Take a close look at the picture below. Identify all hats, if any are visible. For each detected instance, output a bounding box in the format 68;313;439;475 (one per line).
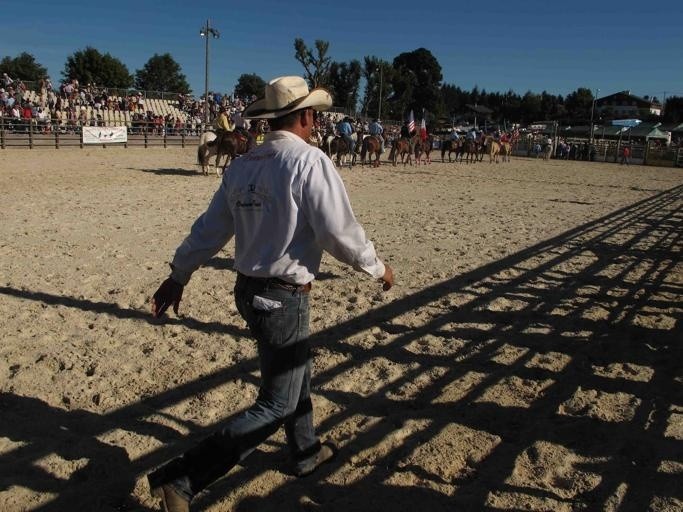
241;75;333;120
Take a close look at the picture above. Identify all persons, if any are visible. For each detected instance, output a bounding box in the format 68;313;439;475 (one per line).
150;75;396;509
1;74;270;153
305;110;682;166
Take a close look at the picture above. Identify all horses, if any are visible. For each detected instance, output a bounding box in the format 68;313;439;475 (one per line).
308;125;324;147
197;119;258;179
440;137;465;163
359;132;389;169
466;133;487;165
387;134;413;168
489;133;512;165
542;138;553;161
414;135;434;165
323;130;361;170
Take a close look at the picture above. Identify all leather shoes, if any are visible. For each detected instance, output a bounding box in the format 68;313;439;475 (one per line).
146;471;189;512
294;439;338;476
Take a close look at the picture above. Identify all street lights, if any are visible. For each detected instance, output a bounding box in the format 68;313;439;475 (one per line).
200;18;219;131
372;59;388;119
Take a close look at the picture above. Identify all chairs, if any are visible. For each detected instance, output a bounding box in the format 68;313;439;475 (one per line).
21;90;186;128
